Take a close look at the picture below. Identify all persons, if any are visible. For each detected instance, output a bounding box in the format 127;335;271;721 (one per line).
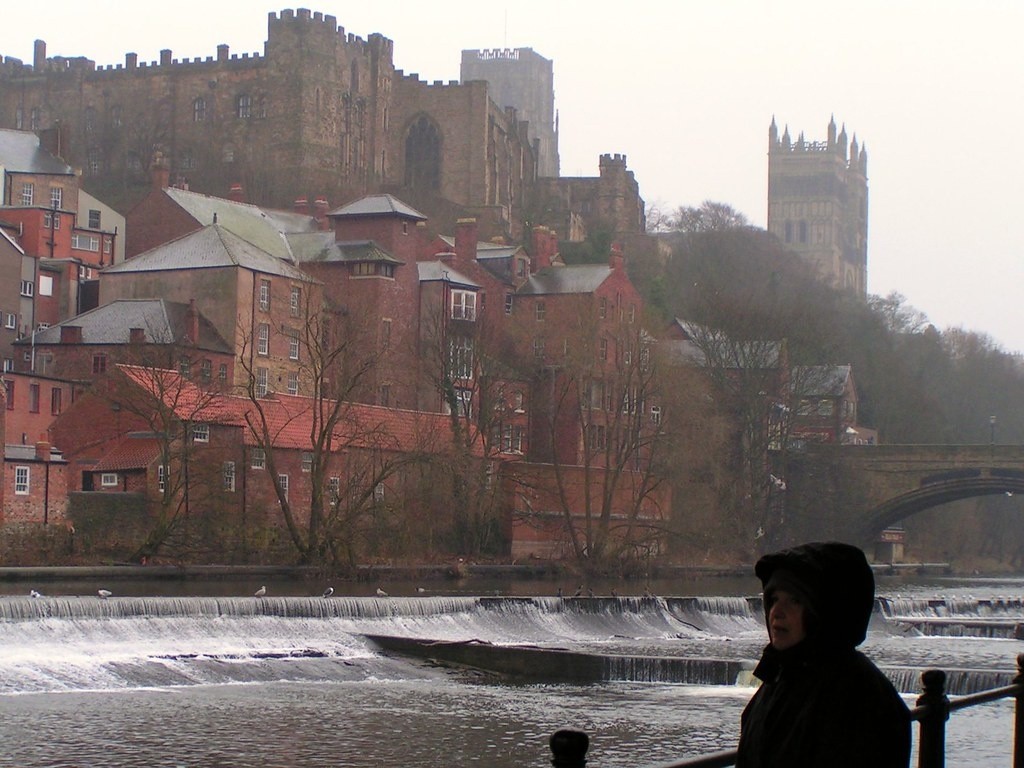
735;541;912;768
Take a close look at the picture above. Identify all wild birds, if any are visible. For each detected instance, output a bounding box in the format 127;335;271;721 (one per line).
969;596;973;600
557;589;564;597
415;588;425;592
323;586;334;598
255;586;266;598
30;589;41;599
897;594;900;599
612;589;620;597
588;588;594;596
910;596;914;599
990;595;1021;600
941;596;945;599
952;595;956;600
377;587;390;598
962;595;966;598
574;584;583;596
98;590;111;599
